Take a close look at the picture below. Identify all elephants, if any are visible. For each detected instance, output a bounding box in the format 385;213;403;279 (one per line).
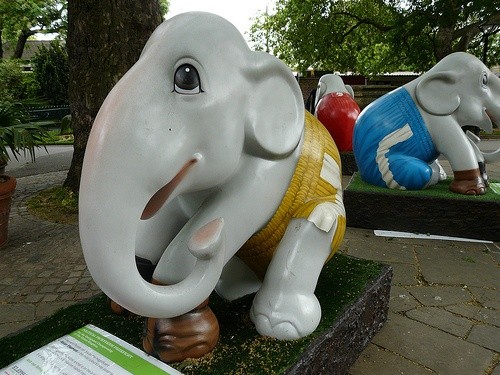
79;11;346;363
353;52;500;195
314;74;360;152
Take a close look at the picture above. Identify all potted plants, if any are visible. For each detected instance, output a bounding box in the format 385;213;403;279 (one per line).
1;93;65;250
